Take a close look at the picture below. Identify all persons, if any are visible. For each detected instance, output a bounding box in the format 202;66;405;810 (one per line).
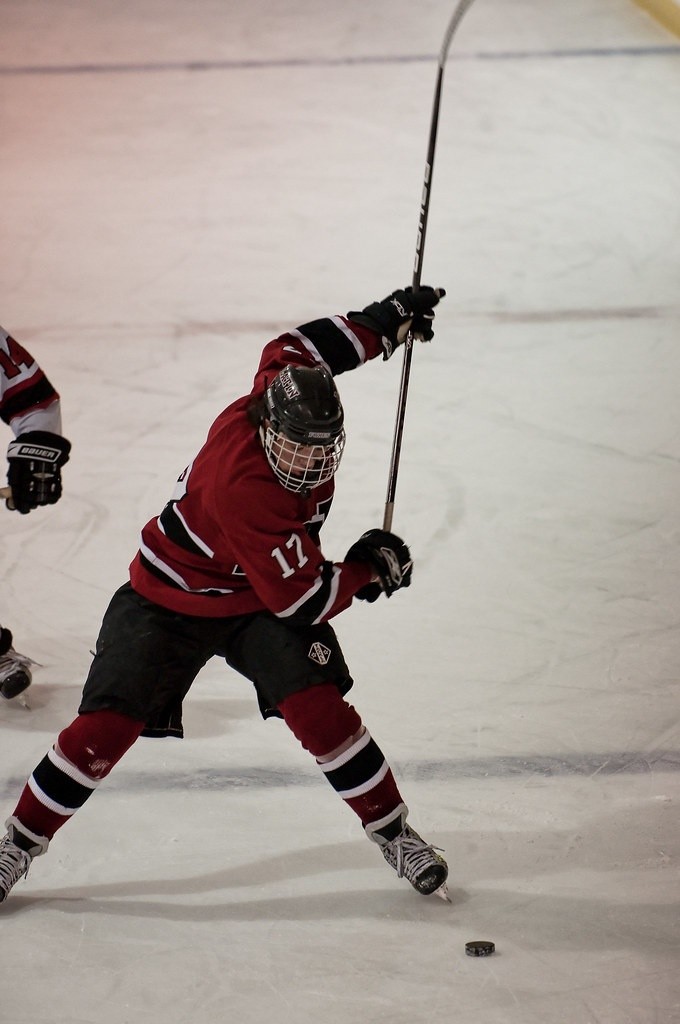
1;284;450;903
0;328;73;710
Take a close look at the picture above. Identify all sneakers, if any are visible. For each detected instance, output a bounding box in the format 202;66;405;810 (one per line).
361;802;452;903
0;817;49;905
0;626;46;713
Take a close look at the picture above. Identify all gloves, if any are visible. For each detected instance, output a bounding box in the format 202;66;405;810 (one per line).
6;430;73;515
344;528;413;603
347;281;446;361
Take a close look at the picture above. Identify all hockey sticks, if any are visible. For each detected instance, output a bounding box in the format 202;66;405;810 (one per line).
383;1;474;532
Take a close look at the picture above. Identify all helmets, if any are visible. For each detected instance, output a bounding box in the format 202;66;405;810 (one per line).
263;364;344;494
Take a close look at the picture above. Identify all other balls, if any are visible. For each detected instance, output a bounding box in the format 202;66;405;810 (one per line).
464;940;496;958
